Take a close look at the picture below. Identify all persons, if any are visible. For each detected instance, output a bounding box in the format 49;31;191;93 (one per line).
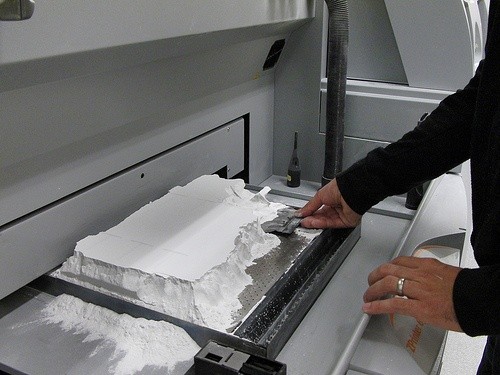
295;0;500;375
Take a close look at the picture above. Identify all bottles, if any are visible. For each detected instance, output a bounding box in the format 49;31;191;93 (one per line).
287;131;301;188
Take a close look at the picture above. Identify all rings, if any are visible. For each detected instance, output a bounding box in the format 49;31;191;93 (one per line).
398;276;406;295
